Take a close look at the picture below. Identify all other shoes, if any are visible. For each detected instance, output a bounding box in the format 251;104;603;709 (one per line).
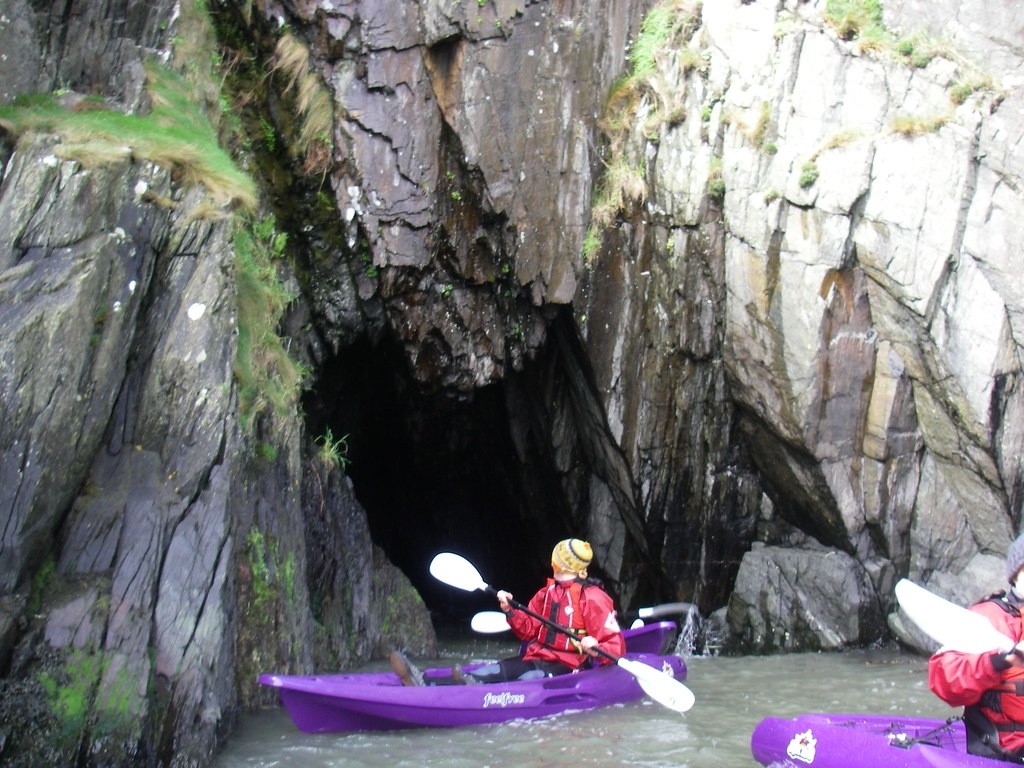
391;651;431;686
453;663;483;686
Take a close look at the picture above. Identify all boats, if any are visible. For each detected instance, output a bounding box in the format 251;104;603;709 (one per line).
257;601;700;731
750;711;1024;768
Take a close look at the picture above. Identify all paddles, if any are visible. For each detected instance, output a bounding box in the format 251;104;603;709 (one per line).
470;600;699;634
894;576;1018;656
429;553;697;714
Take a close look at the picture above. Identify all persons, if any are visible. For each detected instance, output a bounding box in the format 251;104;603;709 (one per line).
927;532;1024;763
389;539;625;685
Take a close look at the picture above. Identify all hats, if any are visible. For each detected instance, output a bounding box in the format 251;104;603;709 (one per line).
1006;534;1024;586
552;539;593;579
